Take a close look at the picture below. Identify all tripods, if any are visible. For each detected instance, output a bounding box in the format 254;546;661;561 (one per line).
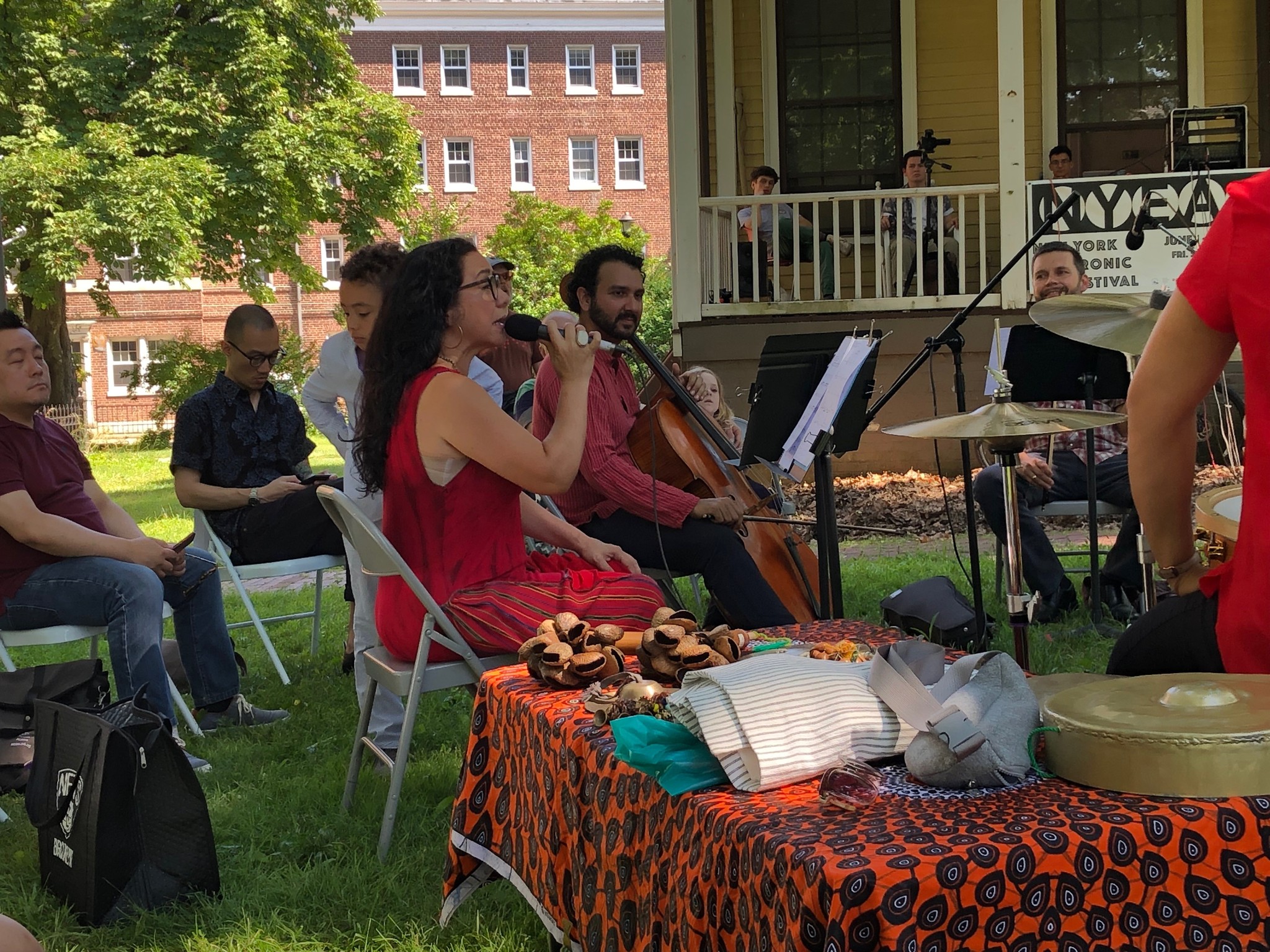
903;150;961;296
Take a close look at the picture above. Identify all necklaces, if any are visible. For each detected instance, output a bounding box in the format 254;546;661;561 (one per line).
439;354;459;370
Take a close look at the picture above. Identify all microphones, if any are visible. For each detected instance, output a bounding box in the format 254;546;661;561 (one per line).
505;314;626;358
1125;190;1152;250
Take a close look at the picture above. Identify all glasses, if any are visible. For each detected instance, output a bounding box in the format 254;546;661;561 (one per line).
227;340;286;368
495;272;514;281
457;274;501;300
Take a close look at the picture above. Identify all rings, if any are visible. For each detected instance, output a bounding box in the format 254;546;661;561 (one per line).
1032;475;1037;479
576;331;589;346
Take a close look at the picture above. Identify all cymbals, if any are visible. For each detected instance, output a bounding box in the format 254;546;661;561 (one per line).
880;392;1128;440
1029;288;1244;362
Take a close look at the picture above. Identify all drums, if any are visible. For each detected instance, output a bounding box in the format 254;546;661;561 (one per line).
1195;480;1244;569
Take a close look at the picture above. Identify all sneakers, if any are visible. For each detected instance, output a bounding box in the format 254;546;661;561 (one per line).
174;735;212;773
199;694;289;731
826;233;852;257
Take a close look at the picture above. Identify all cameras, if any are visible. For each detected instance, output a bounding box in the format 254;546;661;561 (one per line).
916;129;952;151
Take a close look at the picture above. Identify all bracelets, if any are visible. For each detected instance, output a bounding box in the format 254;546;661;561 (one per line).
1157;546;1204;579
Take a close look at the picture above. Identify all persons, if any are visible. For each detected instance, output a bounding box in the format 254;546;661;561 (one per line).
168;304;355;675
301;237;794;759
1049;145;1076;179
880;150;960;298
971;241;1153;625
0;308;291;773
1105;170;1270;678
737;165;853;300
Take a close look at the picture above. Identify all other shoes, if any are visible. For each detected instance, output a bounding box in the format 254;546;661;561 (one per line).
1030;581;1079;626
342;641;355;675
1099;576;1143;622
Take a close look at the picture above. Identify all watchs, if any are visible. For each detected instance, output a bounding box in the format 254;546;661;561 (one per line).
248;487;261;509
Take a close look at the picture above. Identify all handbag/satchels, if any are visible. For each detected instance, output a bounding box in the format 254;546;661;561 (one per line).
876;574;997;651
24;680;226;929
864;631;1039;787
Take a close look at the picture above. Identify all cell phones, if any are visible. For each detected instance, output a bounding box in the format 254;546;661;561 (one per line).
299;475;330;485
166;532;196;560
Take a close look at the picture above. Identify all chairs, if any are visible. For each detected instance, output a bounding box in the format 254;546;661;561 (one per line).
191;508;345;685
744;227;794;301
996;499;1132;606
0;600;205;745
316;486;523;862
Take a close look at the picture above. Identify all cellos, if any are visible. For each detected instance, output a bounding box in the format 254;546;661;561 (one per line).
625;329;833;630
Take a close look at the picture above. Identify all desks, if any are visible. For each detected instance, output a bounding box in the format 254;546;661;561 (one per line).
440;621;1270;952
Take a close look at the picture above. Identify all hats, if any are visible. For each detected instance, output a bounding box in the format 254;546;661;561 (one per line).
486;257;514;271
